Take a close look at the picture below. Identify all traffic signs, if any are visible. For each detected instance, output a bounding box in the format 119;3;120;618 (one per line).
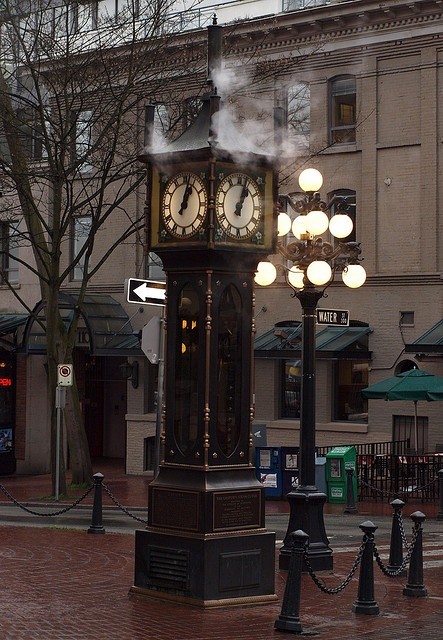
315;308;350;327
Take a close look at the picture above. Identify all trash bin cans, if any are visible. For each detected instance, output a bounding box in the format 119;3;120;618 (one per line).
255;445;281;500
326;445;360;502
281;446;302;500
314;456;327;494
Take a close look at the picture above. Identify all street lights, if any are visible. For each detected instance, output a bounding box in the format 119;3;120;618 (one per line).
254;167;366;572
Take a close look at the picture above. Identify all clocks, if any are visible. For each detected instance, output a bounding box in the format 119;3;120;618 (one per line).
211;158;268;247
146;153;208;245
